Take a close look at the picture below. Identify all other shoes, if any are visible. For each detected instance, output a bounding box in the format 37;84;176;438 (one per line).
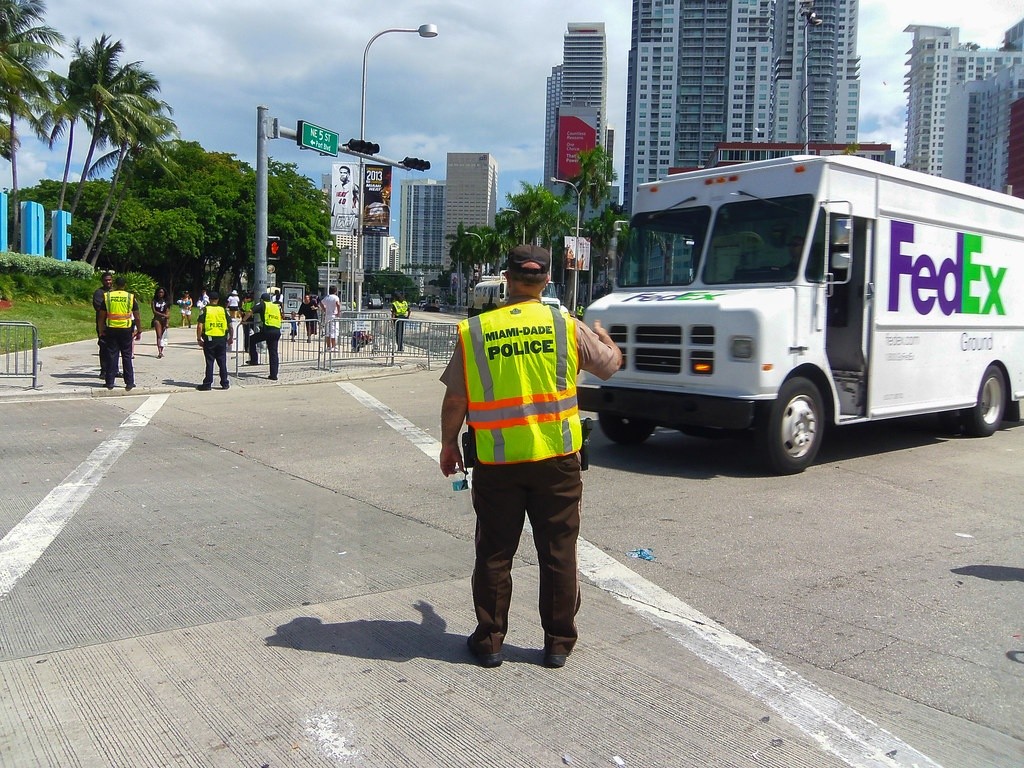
100;374;106;379
115;371;124;378
222;386;229;389
126;384;136;389
103;382;113;388
467;634;502;668
198;385;211;390
246;361;258;365
546;650;572;667
268;375;277;380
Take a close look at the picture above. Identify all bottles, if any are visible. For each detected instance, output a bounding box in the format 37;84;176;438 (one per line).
452;466;472;515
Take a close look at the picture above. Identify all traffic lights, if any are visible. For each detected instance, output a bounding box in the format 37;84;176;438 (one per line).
267;239;288;259
348;138;380;156
403;157;431;172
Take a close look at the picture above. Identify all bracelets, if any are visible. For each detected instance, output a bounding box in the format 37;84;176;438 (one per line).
197;339;201;341
137;331;142;334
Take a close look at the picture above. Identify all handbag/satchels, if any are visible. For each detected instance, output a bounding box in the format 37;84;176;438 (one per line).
159;329;168;347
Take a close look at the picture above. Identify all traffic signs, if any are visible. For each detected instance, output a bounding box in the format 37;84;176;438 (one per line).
296;120;339;157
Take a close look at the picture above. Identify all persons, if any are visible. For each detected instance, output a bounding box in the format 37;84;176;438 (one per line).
179;291;193;328
196;292;233;391
92;272;141;387
577;303;584;320
225;289;263;352
151;286;170;358
320;286;340;352
783;236;818;273
198;289;209;315
439;245;623;668
297;295;319;343
391;293;411;351
288;311;297;342
333;166;360;215
560;304;569;312
243;293;281;380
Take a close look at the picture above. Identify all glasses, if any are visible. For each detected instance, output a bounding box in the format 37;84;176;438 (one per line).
787;242;804;247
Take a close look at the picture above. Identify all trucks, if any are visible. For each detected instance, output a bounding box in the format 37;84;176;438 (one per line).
467;270;561;323
573;152;1024;477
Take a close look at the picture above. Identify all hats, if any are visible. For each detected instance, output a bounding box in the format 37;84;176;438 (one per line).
209;292;218;299
232;290;237;294
505;245;550;274
261;293;270;300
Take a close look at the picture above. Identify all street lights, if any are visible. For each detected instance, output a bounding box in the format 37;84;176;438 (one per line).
549;176;611;318
353;23;439;352
464;231;494;274
499;207;530;245
